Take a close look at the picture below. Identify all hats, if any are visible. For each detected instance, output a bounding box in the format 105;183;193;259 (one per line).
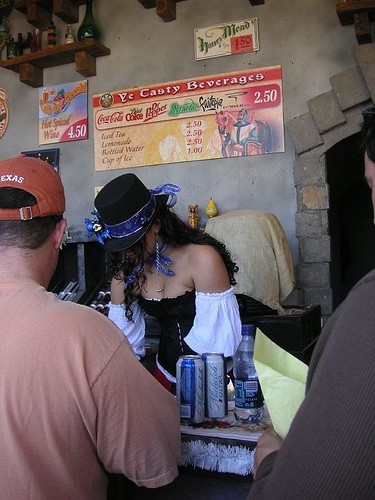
84;173;181;252
0;157;65;221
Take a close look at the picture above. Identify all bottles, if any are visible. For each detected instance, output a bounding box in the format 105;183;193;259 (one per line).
64;24;75;44
77;0;100;41
23;32;32;53
233;325;265;424
15;32;24;56
42;88;49;100
7;38;14;58
52;277;112;316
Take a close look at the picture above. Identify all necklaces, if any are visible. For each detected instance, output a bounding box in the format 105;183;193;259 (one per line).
149;254;175;293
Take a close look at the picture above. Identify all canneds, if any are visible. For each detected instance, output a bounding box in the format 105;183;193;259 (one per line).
202;352;228;418
174;354;205;425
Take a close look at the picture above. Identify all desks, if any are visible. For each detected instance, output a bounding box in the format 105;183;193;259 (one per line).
176;400;276;475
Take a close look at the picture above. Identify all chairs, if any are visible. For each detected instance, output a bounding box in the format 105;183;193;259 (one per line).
204;209;297;307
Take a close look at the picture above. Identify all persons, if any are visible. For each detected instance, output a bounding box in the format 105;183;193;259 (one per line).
85;173;242;394
0;157;182;500
248;123;375;500
